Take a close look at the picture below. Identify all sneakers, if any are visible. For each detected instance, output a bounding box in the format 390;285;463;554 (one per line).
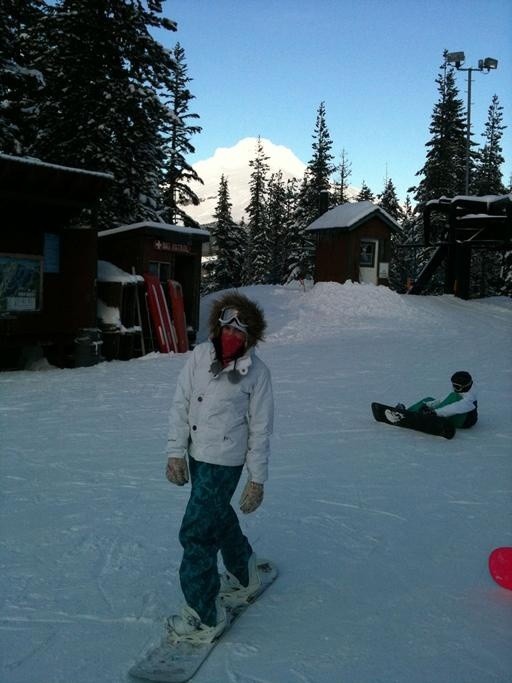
167;598;226;639
396;403;405;410
217;551;261;598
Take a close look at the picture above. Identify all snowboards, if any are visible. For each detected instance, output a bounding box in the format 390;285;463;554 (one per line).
128;557;278;682
371;401;456;440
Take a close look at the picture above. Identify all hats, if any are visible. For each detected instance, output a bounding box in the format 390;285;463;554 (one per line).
220;306;248;347
451;371;472;392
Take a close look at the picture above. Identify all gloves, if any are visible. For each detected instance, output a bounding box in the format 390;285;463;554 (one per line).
166;457;188;486
238;482;263;514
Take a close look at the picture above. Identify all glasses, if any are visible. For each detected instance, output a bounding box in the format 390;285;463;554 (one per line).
218;305;249;328
452;381;472;391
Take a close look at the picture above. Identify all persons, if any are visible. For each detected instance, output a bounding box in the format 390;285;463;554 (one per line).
394;371;478;429
163;294;275;640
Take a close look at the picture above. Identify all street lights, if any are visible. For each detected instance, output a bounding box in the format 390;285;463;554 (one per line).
446;51;498;196
